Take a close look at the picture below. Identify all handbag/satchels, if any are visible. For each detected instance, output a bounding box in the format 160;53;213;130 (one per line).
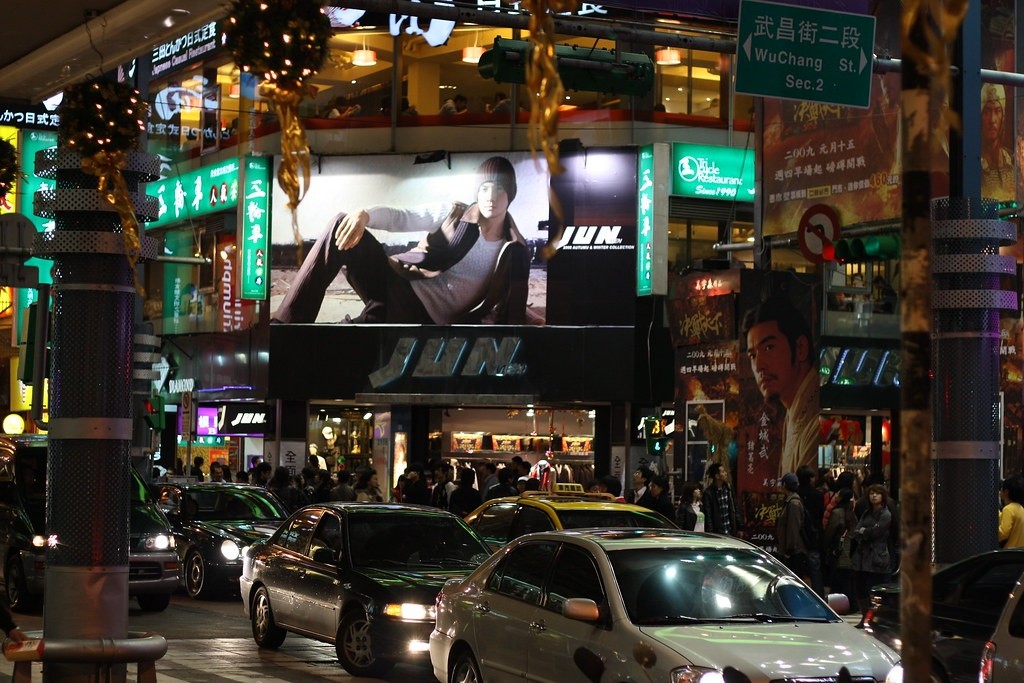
824;543;843;559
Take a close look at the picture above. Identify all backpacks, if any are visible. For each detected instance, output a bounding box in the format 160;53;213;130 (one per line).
787;497;820;550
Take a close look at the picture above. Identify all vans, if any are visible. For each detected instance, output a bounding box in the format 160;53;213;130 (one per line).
0;434;181;613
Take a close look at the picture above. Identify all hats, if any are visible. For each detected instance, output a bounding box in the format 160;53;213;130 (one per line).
518;477;528;482
777;473;798;485
475;156;516;202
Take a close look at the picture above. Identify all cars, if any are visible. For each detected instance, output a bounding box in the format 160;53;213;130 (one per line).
154;482;1024;683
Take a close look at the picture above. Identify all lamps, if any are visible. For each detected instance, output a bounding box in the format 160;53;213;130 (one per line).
413;147;448;167
352;28;377;65
165;7;190;27
462;31;487;63
655;40;680;65
228;83;240;97
559;136;583;150
363;410;373;419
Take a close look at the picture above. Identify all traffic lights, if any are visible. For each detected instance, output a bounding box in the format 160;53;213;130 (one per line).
645;419;665;454
822;236;902;264
143;395;165;431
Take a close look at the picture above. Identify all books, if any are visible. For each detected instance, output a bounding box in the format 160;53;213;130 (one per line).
4;638;45;661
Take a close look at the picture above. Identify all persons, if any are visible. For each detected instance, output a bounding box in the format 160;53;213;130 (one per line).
997;474;1024;549
189;454;384;516
623;461;745;538
272;154;532;327
0;600;29;645
736;299;822;486
152;467;161;483
872;275;896;314
392;455;541;519
585;473;622;501
772;465;900;630
